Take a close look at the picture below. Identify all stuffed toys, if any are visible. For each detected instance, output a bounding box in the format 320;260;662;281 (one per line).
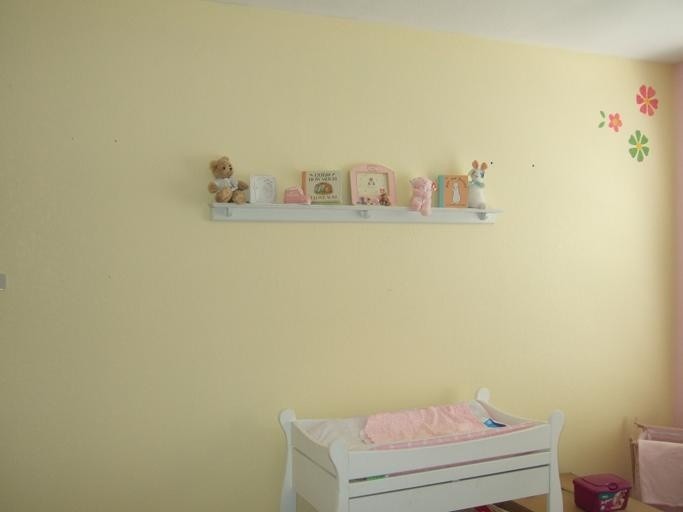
467;159;488;210
207;155;249;204
408;176;438;217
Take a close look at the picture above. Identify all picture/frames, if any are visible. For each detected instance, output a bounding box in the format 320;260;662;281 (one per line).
346;162;398;206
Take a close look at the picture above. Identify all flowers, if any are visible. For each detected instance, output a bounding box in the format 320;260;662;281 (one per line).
598;82;660;166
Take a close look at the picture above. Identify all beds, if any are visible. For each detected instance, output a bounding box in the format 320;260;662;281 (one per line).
278;387;568;512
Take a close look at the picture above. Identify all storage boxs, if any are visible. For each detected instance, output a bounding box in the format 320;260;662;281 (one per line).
569;471;634;510
